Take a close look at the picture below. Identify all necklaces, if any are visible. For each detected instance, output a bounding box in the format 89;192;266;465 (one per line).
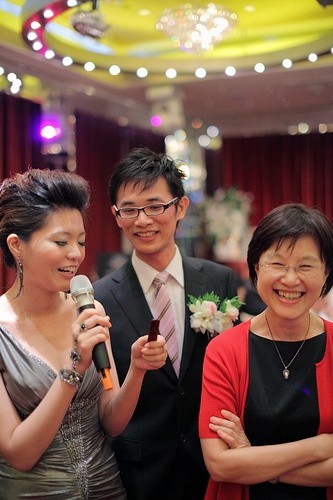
265;308;310;378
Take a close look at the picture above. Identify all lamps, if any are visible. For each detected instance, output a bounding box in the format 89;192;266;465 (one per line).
72;0;109;37
157;8;237;51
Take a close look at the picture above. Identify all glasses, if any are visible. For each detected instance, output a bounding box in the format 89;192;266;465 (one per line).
257;259;325;277
112;196;180;220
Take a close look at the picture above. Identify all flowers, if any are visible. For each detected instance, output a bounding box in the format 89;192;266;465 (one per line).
187;291;246;336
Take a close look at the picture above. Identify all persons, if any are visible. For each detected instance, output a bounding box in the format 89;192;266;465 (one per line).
86;151;237;500
0;169;167;500
198;203;333;500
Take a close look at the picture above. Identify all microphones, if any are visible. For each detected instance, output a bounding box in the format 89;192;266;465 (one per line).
70;274;114;391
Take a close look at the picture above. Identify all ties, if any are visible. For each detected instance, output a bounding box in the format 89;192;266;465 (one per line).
153;271;181;377
